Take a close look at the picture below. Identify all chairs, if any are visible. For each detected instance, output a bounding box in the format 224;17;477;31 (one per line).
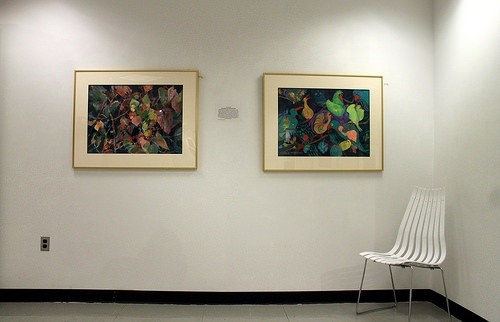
355;183;453;322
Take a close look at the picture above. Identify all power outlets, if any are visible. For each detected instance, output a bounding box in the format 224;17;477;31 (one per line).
40;236;50;250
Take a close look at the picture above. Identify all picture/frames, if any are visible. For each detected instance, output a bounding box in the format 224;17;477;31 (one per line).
261;72;384;172
72;68;200;173
